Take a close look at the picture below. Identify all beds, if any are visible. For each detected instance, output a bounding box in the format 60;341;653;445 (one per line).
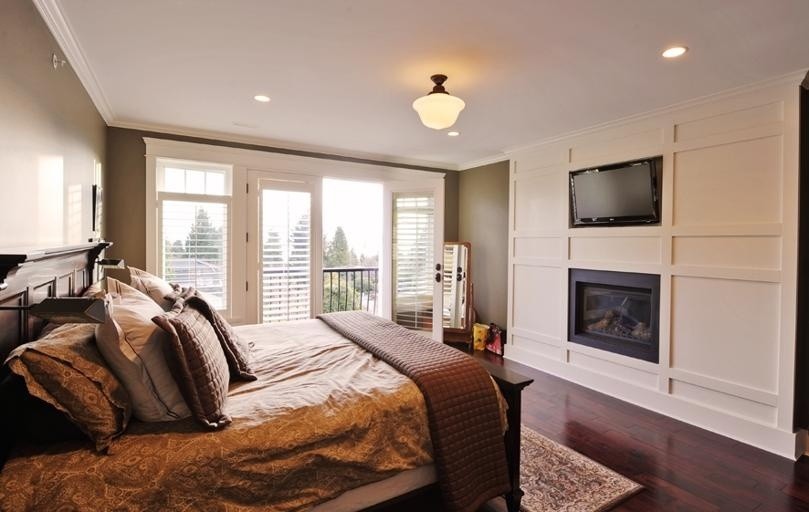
1;241;534;511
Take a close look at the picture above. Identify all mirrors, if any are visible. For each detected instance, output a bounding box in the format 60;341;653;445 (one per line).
442;241;471;333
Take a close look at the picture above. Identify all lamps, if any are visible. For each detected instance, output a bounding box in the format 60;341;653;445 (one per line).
410;75;465;130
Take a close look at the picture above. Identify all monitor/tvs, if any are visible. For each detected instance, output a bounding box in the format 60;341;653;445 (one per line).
569;159;661;226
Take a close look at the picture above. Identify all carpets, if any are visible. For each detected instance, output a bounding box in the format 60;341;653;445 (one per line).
520;425;648;511
